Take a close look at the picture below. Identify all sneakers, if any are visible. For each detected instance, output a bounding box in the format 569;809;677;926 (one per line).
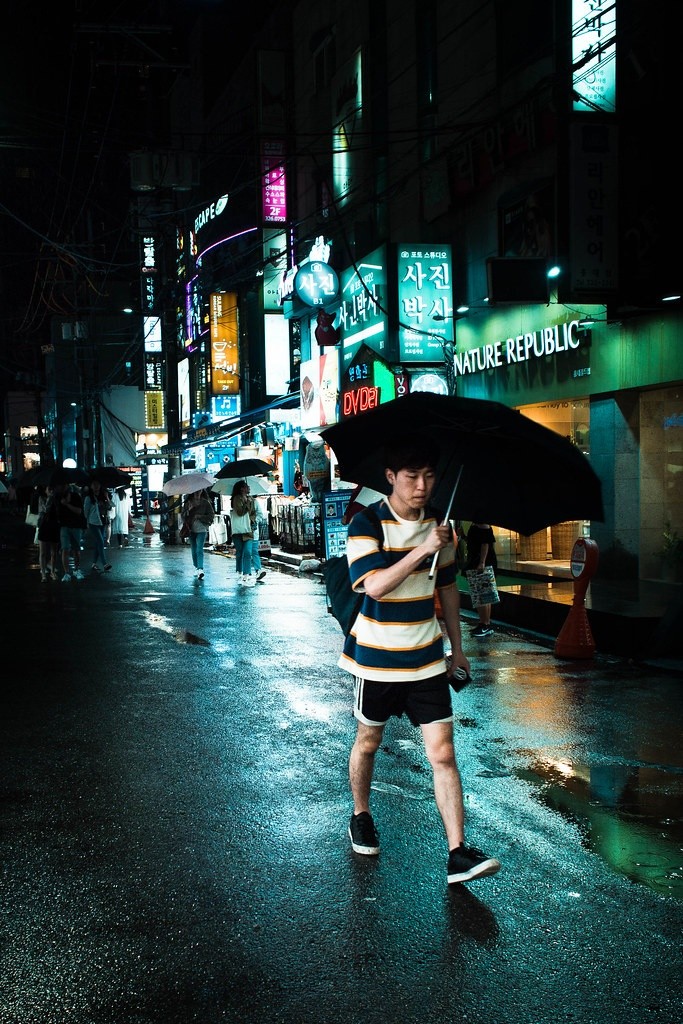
472;622;494;636
348;812;380;855
447;842;501;884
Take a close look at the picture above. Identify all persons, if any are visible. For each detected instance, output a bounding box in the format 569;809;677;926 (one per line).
458;522;501;636
230;480;267;587
0;483;23;512
347;447;499;885
327;504;335;516
181;489;214;580
25;483;87;582
84;479;111;571
98;481;134;548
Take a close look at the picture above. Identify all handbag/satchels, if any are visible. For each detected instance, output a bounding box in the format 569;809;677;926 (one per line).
179;523;189;537
107;507;116;519
230;510;251;534
466;565;501;608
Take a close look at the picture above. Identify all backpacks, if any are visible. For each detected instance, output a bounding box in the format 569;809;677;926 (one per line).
319;508;384;637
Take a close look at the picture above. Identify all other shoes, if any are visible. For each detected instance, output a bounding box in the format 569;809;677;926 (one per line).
194;567;198;577
198;569;204;578
61;574;71;582
72;571;84;579
50;573;58;580
105;564;112;570
92;564;100;570
119;545;123;548
236;570;266;587
41;575;47;582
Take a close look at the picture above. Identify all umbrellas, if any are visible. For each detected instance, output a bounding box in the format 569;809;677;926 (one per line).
77;467;133;488
0;480;9;495
214;459;278;483
162;473;215;497
211;476;273;496
319;391;607;576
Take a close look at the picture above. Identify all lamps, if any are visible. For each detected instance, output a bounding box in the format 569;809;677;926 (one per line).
433;314;464;320
